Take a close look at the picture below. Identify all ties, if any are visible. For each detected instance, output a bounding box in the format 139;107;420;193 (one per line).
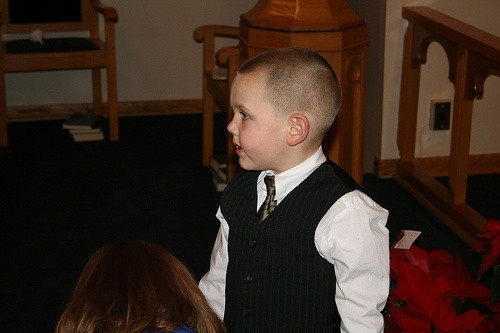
257;175;277;224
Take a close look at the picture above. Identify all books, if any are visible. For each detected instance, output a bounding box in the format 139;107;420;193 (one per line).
208;154;228;191
62;113;109;142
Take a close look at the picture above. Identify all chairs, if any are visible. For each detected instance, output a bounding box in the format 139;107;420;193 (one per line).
194;0;368;189
0;0;121;138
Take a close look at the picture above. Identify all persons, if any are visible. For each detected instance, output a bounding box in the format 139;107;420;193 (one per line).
197;46;390;333
56;241;226;333
